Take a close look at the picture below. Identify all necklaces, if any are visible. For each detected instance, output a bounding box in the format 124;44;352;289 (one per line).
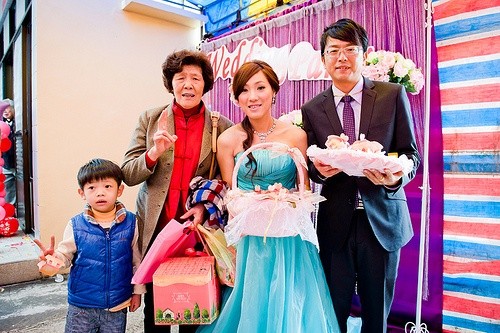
253;118;276;143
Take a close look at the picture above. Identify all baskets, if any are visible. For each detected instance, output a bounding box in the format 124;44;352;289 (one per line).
226;142;305;237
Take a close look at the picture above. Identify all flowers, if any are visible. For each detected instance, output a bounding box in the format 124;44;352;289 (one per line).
278;109;303;129
363;50;424;96
246;183;300;244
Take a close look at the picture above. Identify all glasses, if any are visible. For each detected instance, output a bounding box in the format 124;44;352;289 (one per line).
322;46;364;57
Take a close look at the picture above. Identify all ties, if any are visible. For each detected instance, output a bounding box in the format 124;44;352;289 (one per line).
340;95;359;208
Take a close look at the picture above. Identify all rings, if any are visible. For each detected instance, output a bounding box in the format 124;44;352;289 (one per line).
380;176;384;181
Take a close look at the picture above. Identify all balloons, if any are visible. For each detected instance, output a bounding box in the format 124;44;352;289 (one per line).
0;120;19;237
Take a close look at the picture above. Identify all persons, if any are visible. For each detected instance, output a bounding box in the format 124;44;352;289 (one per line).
120;51;235;333
3;99;18;218
197;60;341;333
300;18;420;333
34;159;147;333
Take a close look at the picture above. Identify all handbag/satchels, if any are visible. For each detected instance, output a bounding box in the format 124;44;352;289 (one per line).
132;219;238;325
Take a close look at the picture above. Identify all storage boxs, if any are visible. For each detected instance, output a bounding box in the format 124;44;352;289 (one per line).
152;255;218;325
